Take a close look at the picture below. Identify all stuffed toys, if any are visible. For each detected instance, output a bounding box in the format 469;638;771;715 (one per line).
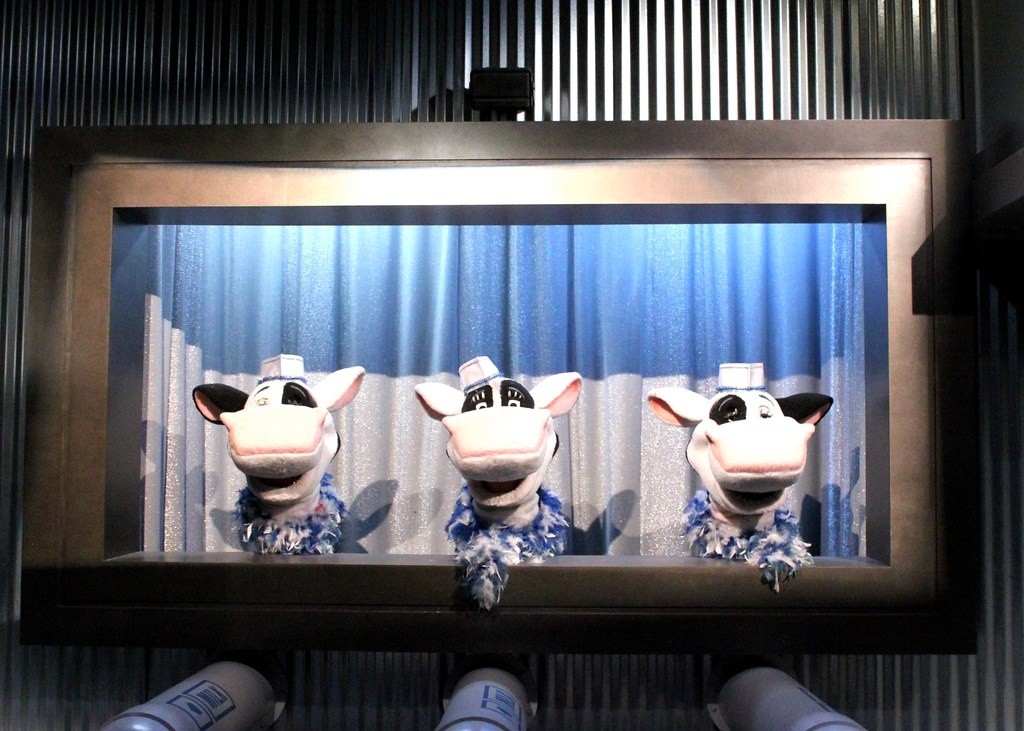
191;353;368;555
413;356;584;607
645;361;833;580
